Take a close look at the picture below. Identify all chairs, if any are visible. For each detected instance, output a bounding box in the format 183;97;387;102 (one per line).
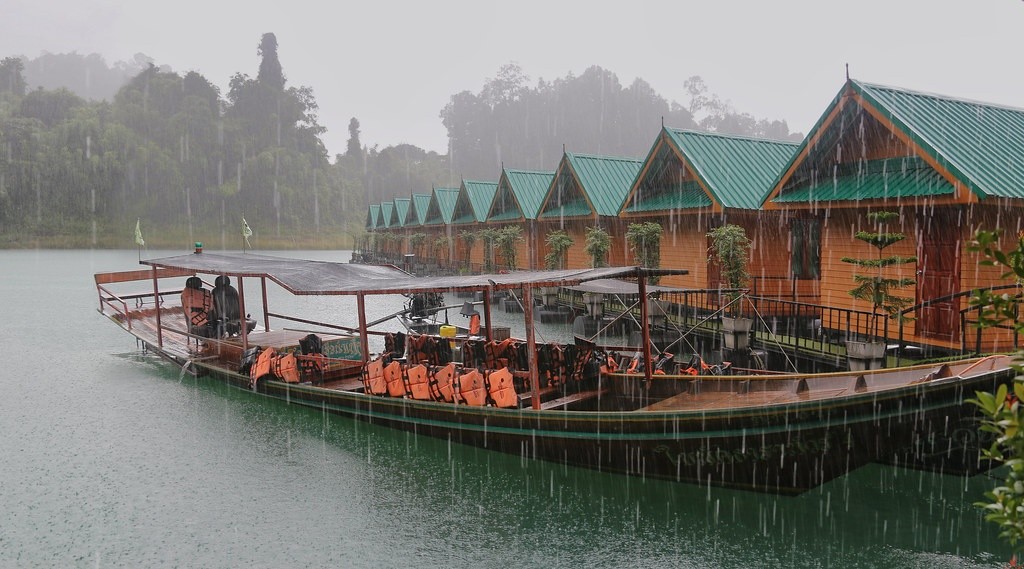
181;275;256;345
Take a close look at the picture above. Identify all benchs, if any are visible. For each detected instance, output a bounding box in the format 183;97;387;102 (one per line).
303;363;363;391
497;383;606;410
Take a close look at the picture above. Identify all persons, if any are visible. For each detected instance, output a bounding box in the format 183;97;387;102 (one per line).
468;315;481;337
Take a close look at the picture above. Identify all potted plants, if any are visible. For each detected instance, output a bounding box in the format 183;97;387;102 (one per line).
705;223;753;348
351;225;525;297
540;226;575;306
846;211;919;372
626;220;664;324
582;226;612;318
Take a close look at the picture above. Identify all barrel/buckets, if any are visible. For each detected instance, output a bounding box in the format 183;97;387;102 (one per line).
440;325;456;348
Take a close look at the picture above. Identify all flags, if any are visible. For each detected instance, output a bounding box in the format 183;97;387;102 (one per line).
135;218;144;246
240;216;252;248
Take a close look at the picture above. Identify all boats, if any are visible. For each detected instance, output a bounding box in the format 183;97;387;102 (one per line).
91;210;1024;499
